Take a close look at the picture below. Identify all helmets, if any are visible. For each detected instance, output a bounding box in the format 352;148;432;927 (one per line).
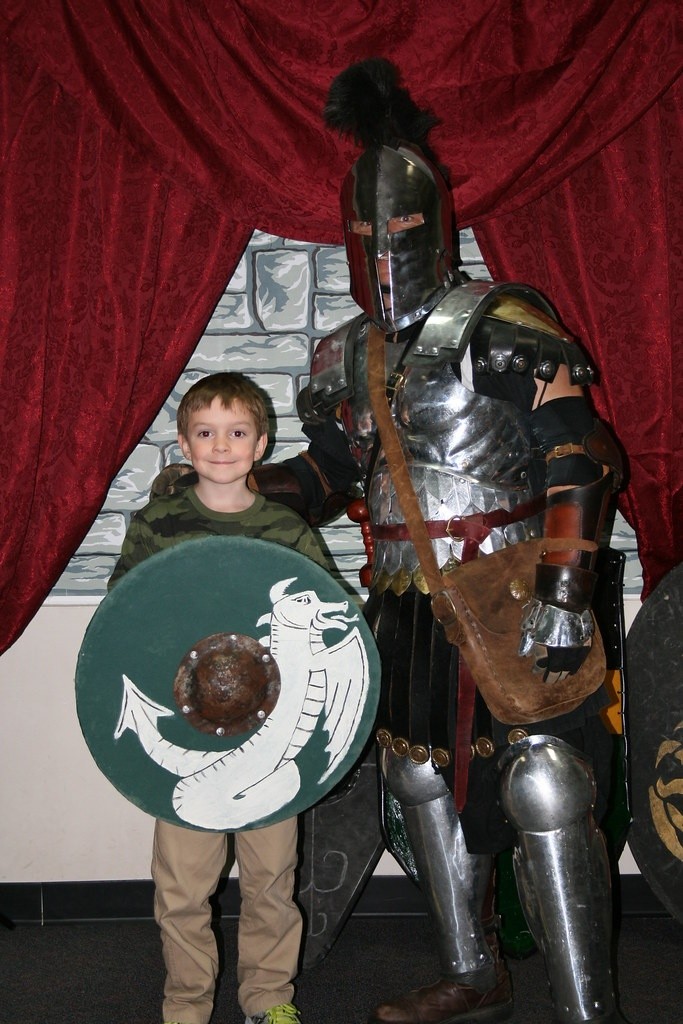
326;60;455;334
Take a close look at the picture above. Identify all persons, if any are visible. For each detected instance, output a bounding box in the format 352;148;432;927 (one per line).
107;371;339;1024
149;57;628;1024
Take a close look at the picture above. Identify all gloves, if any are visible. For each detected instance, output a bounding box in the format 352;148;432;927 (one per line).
519;563;600;685
149;463;199;502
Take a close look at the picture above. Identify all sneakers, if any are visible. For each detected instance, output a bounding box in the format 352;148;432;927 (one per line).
245;1003;301;1024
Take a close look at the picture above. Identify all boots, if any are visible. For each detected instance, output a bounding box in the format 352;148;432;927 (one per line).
367;853;515;1024
